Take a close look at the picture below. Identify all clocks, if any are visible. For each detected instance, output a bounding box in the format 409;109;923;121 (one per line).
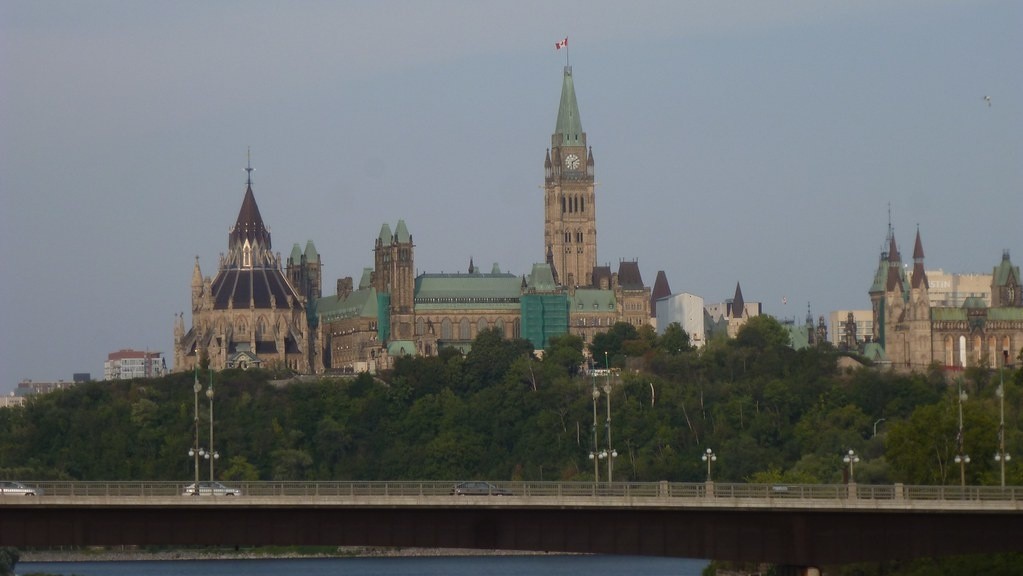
565;154;581;170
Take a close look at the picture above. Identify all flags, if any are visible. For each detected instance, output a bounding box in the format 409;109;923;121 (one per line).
555;37;568;51
779;294;790;305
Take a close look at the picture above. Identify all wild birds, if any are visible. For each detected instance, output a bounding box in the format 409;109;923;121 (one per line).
783;295;788;305
984;95;992;107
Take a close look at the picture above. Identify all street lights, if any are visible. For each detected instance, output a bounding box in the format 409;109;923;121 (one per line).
954;360;971;500
994;356;1011;497
700;448;717;498
590;352;618;495
188;358;219;496
842;449;860;500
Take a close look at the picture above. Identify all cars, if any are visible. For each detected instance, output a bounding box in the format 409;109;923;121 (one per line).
451;481;515;496
0;480;44;496
181;480;242;498
